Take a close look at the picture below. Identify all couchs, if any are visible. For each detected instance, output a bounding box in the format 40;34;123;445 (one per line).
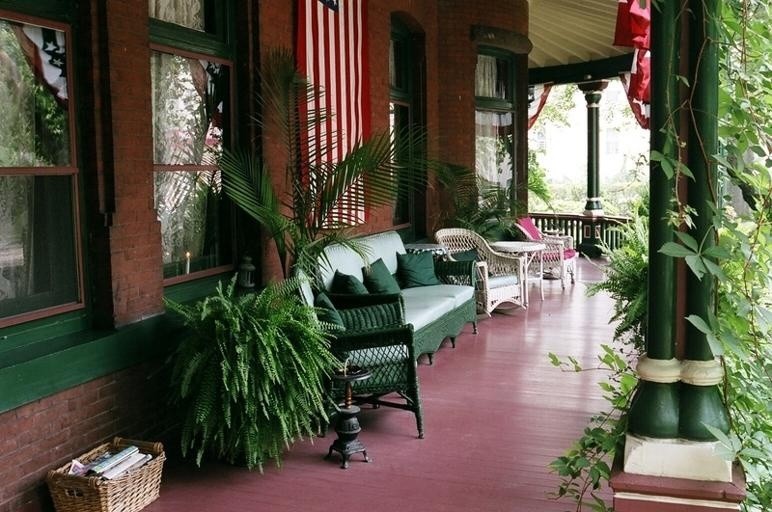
315;230;479;368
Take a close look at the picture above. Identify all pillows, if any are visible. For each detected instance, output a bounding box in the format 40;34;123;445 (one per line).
315;292;349;361
517;218;539;239
332;269;368;294
397;253;438;289
363;258;400;294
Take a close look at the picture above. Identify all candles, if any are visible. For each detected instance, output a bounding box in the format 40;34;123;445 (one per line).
185;252;190;274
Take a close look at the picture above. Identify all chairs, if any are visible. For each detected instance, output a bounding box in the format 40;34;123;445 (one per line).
297;269;425;440
435;227;526;317
515;221;576;289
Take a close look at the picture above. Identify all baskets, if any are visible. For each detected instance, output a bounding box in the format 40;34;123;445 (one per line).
46;437;166;512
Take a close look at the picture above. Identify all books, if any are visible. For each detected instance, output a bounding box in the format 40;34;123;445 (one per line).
67;443;154;484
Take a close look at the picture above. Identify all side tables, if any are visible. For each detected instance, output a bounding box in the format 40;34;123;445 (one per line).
489;241;545;305
324;366;373;468
403;243;456;261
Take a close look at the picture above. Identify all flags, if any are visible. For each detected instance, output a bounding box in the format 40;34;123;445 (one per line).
294;0;371;230
474;110;515;139
185;57;227;130
527;84;553;132
613;2;652;130
7;20;70;108
